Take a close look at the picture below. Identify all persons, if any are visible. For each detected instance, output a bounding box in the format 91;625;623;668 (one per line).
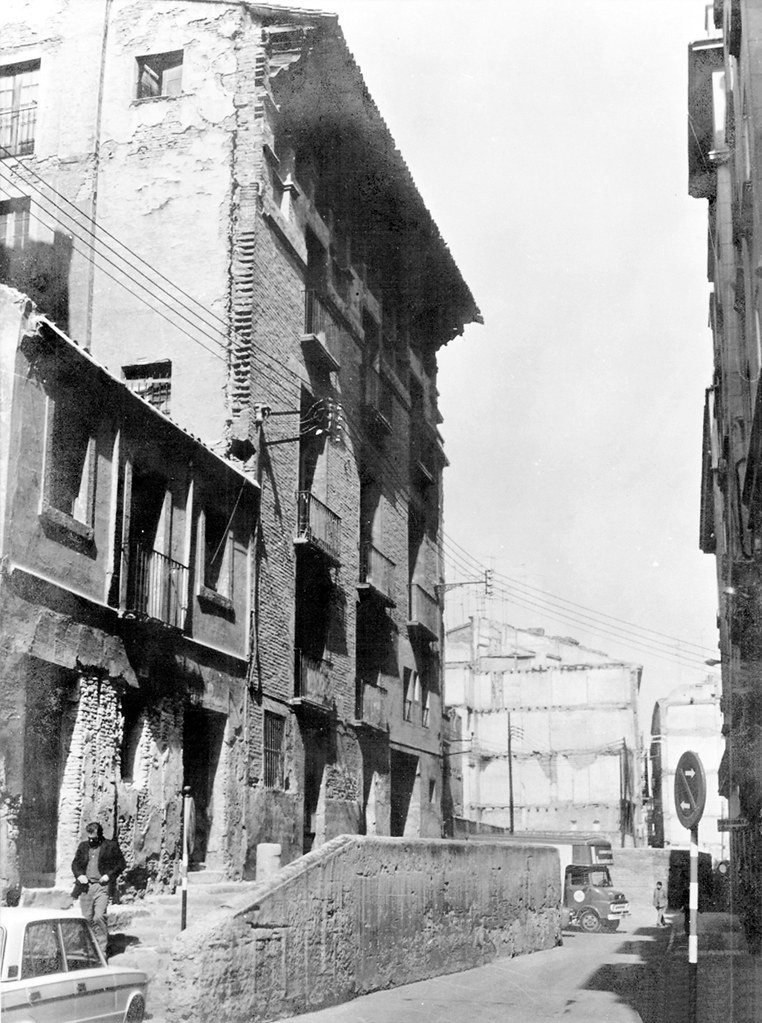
680;901;690;936
653;881;668;926
71;822;127;959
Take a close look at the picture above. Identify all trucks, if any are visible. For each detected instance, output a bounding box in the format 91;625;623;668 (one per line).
465;834;633;933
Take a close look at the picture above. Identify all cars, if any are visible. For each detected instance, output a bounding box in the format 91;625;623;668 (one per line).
0;906;152;1023
